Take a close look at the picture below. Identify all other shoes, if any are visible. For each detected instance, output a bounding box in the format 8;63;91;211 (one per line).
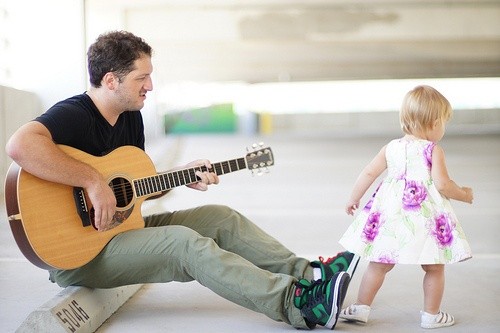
420;309;455;329
340;303;370;324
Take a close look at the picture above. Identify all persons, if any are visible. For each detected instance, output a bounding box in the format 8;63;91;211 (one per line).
339;84;473;329
6;30;360;328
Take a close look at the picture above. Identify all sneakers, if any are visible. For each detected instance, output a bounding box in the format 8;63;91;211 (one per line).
292;249;361;330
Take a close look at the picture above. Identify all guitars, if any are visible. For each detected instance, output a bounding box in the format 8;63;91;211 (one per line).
5;144;274;270
